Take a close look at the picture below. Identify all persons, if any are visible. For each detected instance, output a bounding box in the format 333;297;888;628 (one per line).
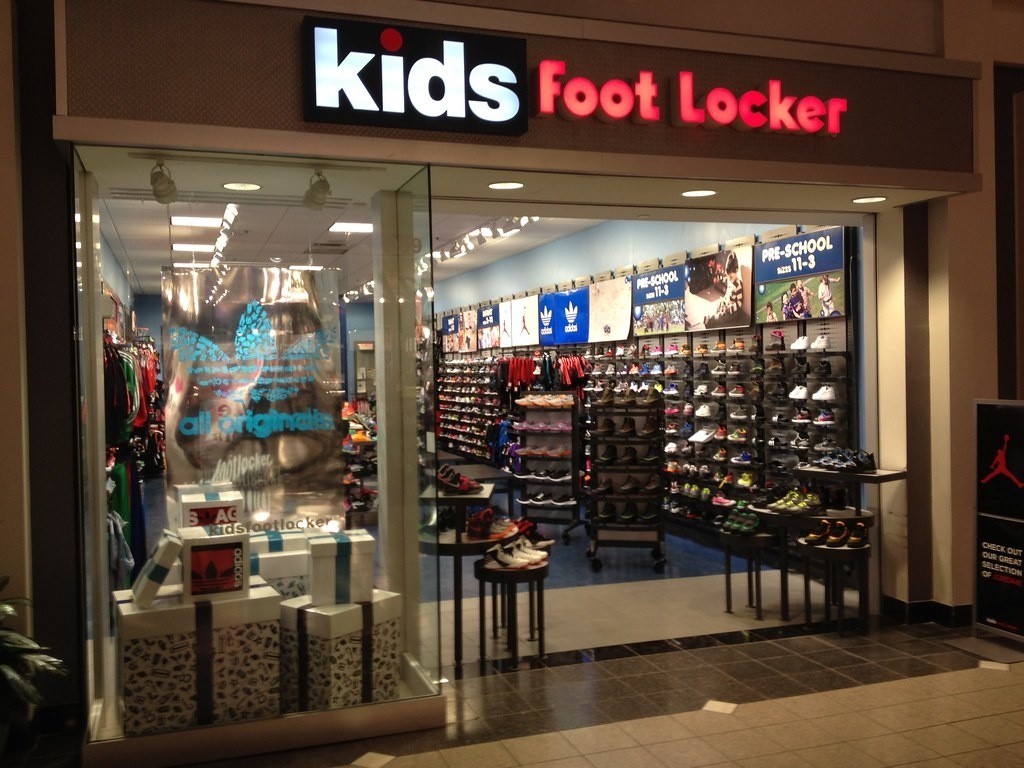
766;302;779;322
781;292;803;321
796;279;814;317
702;253;743;328
446;312;498;353
789;283;805;319
632;298;685;334
818;274;841;317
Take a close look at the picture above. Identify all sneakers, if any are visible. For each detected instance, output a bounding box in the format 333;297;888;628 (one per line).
341;335;875;570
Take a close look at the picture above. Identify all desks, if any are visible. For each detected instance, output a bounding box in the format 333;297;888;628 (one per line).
437;450;467;466
426;462;516;517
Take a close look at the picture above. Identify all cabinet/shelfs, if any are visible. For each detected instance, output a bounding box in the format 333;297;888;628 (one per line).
419;341;911;668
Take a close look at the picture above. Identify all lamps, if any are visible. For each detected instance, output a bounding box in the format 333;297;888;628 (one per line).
303;170;332;209
150;157;176;204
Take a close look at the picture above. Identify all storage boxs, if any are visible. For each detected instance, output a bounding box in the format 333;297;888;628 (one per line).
112;484;400;738
